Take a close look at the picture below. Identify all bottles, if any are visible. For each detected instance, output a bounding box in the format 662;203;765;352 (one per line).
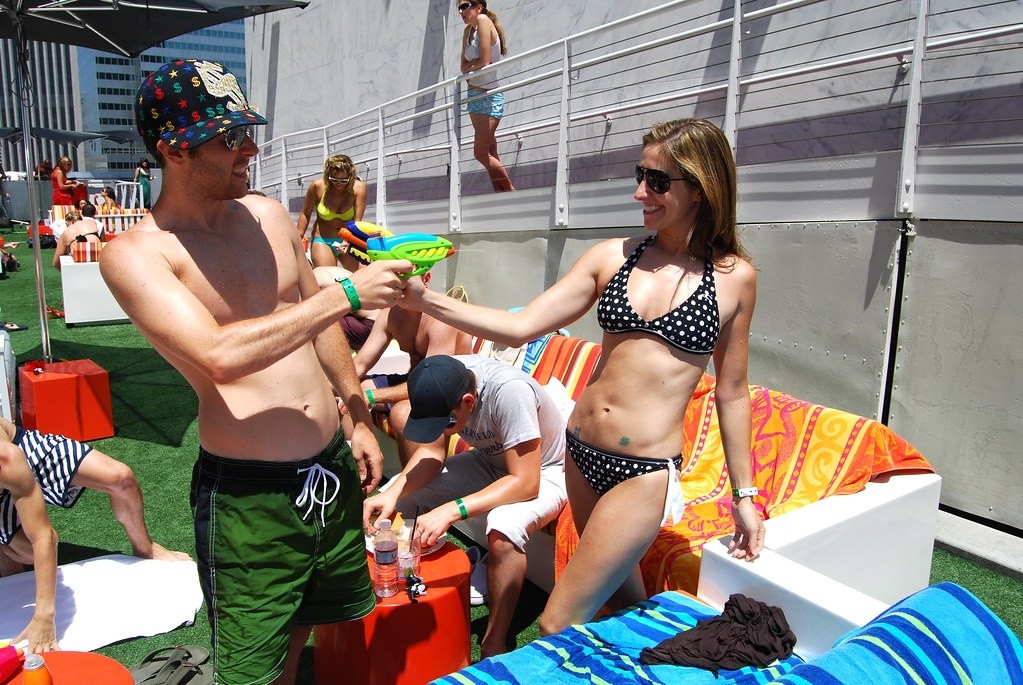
23;653;53;685
373;519;398;597
105;230;117;242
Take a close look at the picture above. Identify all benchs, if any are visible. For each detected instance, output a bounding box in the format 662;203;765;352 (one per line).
79;208;150;235
426;586;1023;685
366;334;939;619
49;205;118;225
58;242;132;325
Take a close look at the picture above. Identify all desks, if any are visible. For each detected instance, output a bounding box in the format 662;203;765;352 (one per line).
311;541;472;685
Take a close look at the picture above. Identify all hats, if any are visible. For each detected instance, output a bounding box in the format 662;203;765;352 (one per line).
135;59;268;151
403;354;471;443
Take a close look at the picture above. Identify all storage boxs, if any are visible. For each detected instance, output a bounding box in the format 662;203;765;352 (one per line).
17;358;115;445
27;225;52;238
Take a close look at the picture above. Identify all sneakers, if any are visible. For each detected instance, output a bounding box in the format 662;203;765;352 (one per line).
464;545;491;606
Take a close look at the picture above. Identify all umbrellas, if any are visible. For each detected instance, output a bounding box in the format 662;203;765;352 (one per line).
0;0;311;362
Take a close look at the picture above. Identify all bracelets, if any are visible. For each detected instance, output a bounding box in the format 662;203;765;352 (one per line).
335;278;362;311
731;487;760;498
366;390;376;406
455;498;468;521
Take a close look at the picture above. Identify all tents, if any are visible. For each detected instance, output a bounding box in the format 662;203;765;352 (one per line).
83;130;141;170
0;126;107;218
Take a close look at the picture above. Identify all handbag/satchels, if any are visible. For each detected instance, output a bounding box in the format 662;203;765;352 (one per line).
40;235;56;249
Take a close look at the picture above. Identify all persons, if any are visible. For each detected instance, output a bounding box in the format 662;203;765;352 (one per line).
333;272;473;469
134;158;154;211
35;159;52;175
0;414;193;655
363;355;568;662
307;259;382;350
97;59;412;685
455;0;517;193
296;155;366;272
51;155;116;272
0;235;19;280
396;118;766;638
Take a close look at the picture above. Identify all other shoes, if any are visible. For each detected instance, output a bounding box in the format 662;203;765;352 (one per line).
46;304;64;319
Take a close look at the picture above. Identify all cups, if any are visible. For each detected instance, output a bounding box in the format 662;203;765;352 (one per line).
392;528;422;578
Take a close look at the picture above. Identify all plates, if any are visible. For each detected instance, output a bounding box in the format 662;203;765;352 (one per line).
365;519;447;556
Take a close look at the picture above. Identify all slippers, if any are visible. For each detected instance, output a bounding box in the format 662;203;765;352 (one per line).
0;321;28;332
127;644;216;685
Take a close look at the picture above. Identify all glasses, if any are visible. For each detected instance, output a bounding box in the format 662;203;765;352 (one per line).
102;190;104;192
223;125;253;151
635;164;688;194
458;2;473;13
445;413;457;428
329;173;351;185
